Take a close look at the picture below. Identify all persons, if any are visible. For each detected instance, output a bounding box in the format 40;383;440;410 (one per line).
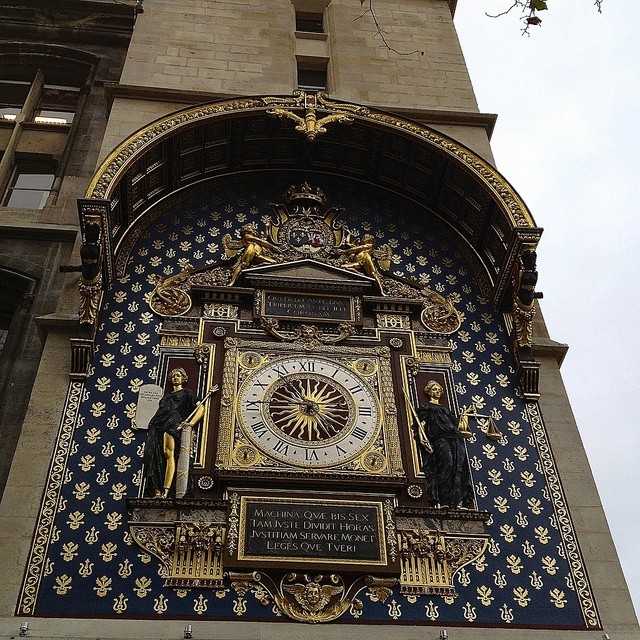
228;224;276;288
404;380;477;510
143;366;204;499
341;233;387;296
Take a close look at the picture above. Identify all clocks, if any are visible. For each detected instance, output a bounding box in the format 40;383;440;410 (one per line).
216;337;406;477
